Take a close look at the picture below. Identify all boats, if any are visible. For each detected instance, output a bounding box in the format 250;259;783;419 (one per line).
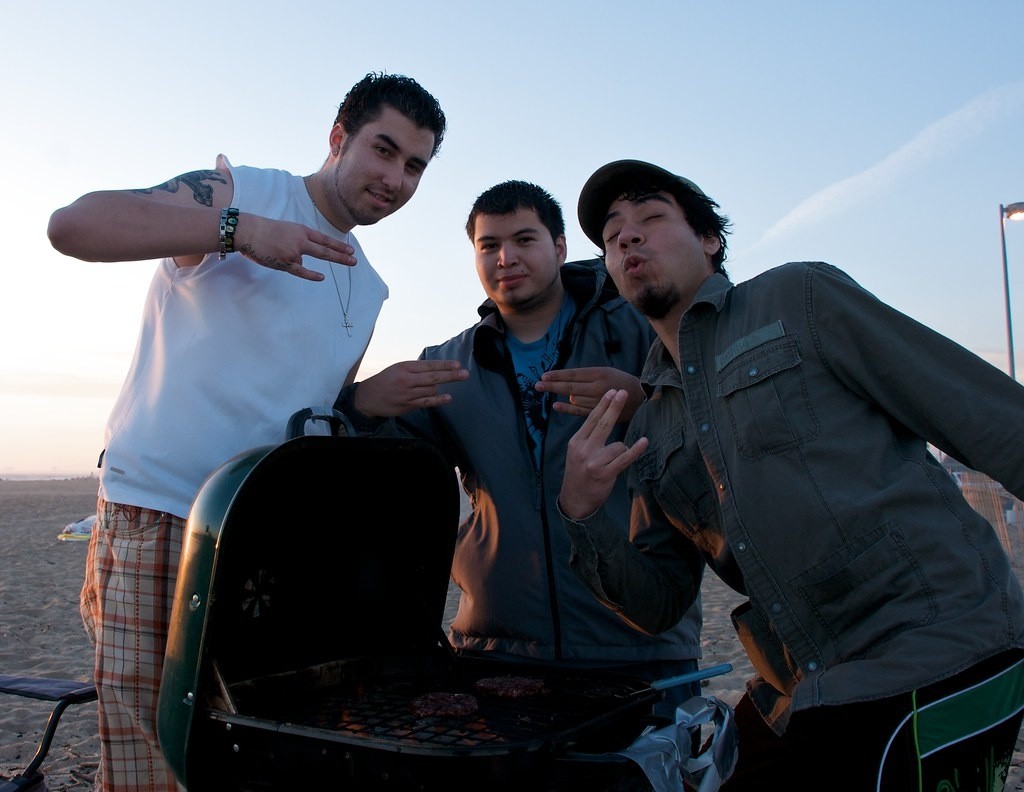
57;516;97;542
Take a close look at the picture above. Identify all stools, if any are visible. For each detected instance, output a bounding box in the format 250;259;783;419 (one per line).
0;675;100;792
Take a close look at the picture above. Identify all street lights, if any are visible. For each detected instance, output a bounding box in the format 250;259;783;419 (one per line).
999;202;1023;380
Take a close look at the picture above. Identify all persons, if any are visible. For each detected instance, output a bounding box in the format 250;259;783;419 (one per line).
45;72;446;792
554;154;1024;792
332;181;702;721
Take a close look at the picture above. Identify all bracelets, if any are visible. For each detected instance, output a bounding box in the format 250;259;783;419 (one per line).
226;208;240;254
218;208;229;261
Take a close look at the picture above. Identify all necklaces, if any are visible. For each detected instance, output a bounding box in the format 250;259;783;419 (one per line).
308;172;354;337
